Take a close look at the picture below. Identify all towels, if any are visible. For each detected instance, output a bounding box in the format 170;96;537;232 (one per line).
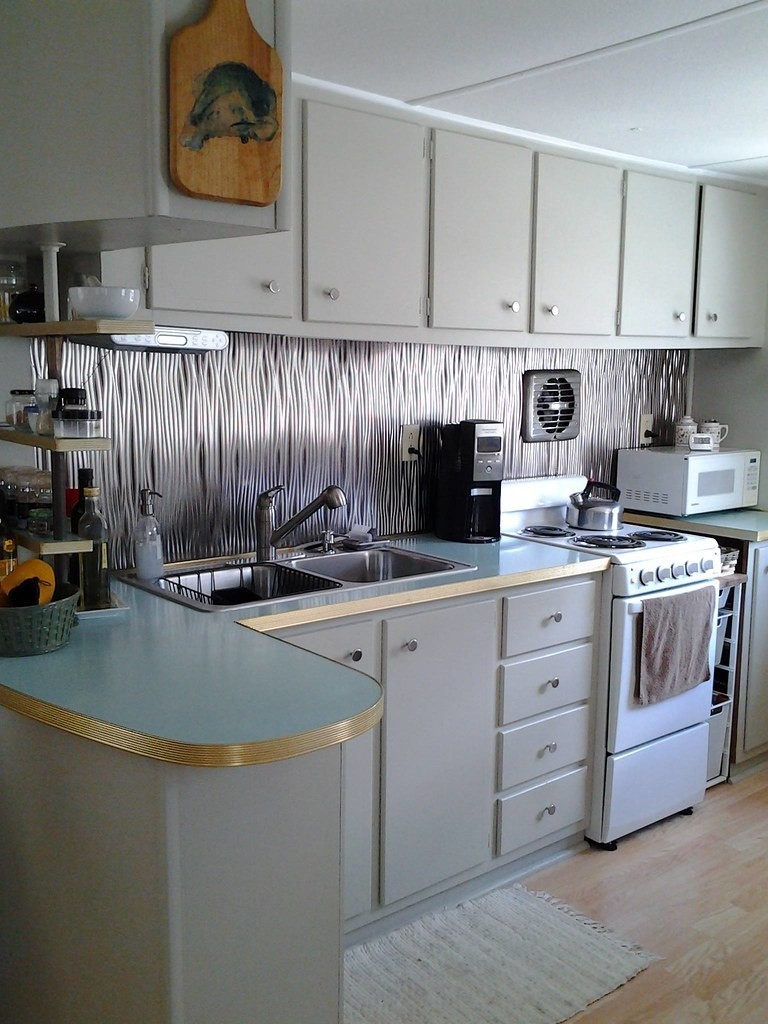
633;585;720;707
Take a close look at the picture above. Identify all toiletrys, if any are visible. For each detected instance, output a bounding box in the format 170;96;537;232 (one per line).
133;488;168;582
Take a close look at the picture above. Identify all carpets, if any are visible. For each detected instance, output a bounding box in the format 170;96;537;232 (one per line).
343;884;665;1024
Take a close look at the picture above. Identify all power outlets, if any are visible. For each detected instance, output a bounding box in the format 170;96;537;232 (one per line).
403;424;421;462
640;413;654;444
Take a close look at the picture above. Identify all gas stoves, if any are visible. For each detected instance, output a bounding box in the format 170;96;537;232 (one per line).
497;475;722;599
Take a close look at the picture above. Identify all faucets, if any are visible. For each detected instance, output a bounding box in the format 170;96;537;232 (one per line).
253;481;349;565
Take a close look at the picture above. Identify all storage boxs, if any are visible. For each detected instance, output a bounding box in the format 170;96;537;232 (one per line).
715;610;732;666
706;692;733;782
51;410;102;439
718;587;732;608
7;283;61;323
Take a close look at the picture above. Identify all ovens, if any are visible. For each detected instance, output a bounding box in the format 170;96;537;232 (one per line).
601;584;721;755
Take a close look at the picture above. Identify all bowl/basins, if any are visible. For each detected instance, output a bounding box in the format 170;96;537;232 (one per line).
68;285;143;322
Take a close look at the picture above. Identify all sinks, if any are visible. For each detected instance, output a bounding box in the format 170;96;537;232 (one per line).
288;540;480;592
161;562;346;617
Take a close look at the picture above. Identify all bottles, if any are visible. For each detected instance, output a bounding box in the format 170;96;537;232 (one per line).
35;373;61;436
68;462;111;614
1;464;59;542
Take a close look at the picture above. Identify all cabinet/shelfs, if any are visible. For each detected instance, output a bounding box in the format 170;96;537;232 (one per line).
0;240;155;583
263;567;602;933
65;83;426;342
732;540;768;765
617;165;764;349
429;121;623;348
0;1;298;254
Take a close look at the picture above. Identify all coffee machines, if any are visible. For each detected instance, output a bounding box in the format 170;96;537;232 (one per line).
428;417;508;543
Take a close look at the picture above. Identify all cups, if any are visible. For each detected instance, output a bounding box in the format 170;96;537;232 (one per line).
674;415;729;447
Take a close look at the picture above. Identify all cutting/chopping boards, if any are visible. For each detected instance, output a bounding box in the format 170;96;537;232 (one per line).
166;0;285;208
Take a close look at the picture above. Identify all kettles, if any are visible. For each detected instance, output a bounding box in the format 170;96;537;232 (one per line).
565;479;625;532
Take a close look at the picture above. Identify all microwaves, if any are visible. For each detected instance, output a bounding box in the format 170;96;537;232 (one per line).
613;441;762;523
